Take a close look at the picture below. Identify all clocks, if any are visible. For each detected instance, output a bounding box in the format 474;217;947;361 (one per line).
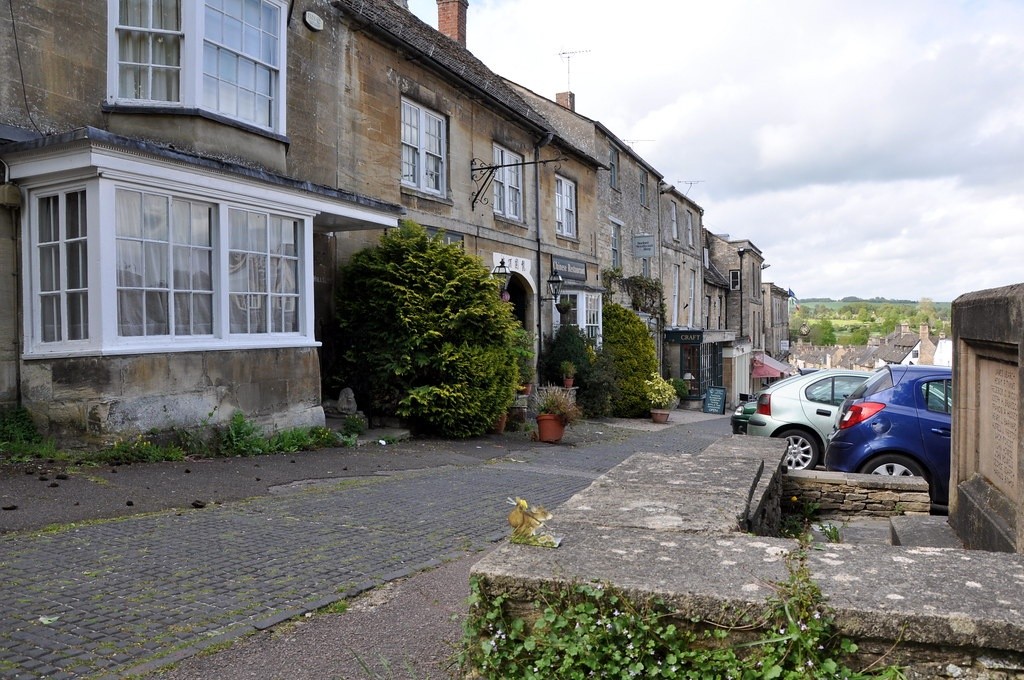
799;323;811;336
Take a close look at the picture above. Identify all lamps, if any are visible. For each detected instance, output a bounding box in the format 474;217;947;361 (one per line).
490;259;512;291
547;269;565;303
683;373;695;390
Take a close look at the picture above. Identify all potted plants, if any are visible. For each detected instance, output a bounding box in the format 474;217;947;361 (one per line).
521;364;535;394
555;298;574;314
535;380;579;445
643;372;688;424
559;359;578;388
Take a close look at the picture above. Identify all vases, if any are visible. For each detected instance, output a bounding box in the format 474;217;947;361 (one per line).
497;415;506;433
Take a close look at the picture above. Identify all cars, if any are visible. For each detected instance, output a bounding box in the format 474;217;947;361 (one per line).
731;366;953;472
824;364;952;503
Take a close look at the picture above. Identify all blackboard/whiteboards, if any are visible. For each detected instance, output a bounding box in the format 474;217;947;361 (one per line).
703;386;727;414
